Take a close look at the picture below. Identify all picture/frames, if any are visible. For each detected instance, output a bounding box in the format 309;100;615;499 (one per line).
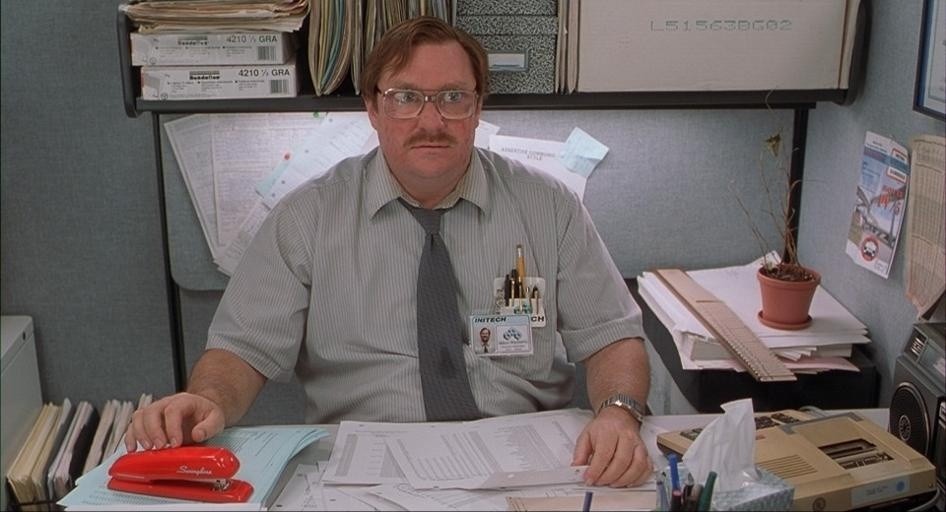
912;0;946;125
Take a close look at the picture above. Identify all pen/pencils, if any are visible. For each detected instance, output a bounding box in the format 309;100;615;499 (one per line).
656;454;717;512
504;245;539;314
583;491;593;512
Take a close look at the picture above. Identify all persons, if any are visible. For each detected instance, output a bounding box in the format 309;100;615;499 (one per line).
125;17;654;488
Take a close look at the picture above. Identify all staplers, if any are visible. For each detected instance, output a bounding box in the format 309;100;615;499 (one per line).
107;446;253;502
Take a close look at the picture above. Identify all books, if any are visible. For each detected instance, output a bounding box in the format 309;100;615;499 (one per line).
10;394;154;511
120;0;456;99
636;246;871;383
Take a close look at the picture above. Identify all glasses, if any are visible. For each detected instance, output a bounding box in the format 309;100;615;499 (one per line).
376;88;476;120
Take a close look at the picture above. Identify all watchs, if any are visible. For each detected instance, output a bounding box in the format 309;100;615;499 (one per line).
590;392;648;426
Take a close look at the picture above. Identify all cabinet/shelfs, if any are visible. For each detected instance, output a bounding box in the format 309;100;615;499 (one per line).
0;315;44;512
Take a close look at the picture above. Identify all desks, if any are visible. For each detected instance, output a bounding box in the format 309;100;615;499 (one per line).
623;276;883;415
226;407;894;511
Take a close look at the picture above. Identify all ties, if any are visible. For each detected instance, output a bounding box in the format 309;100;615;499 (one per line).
396;197;482;420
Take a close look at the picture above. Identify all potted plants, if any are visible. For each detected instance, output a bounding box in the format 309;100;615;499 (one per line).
726;81;824;331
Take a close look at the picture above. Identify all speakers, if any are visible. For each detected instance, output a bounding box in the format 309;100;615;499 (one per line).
887;356;945;463
